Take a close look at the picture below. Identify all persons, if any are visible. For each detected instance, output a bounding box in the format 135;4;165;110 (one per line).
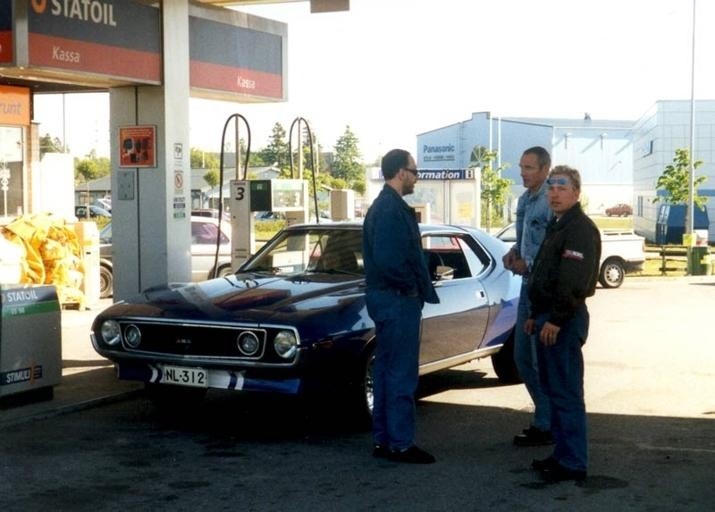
364;149;440;464
512;164;602;486
502;146;555;448
321;250;338;272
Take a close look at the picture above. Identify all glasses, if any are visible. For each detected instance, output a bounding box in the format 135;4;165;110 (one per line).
544;177;576;190
404;167;418;176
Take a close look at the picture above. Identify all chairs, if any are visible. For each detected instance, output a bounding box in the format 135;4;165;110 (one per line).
325;247;358;271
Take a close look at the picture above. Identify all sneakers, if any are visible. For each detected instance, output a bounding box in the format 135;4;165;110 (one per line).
372;444;386;458
513;423;557;448
524;461;588;484
385;444;435;464
532;453;557;470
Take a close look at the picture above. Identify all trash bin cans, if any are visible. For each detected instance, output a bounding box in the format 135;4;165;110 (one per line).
691;246;709;275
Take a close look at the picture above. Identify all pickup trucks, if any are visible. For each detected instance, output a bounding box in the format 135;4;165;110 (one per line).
495;222;645;287
